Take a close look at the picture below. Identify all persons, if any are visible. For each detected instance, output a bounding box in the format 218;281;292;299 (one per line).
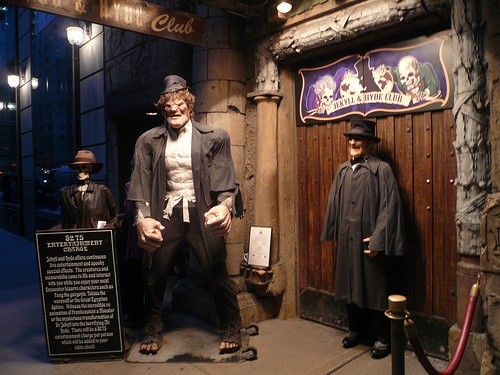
49;150;124;230
321;119;407;356
126;75;243;352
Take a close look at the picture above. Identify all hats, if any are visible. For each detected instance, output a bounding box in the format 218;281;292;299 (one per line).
65;150;103;174
159;74;191;94
343;119;381;144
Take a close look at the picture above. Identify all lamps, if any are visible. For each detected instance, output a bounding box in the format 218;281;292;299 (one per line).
276;0;295;13
7;62;25;88
65;18;92;46
0;96;12;111
